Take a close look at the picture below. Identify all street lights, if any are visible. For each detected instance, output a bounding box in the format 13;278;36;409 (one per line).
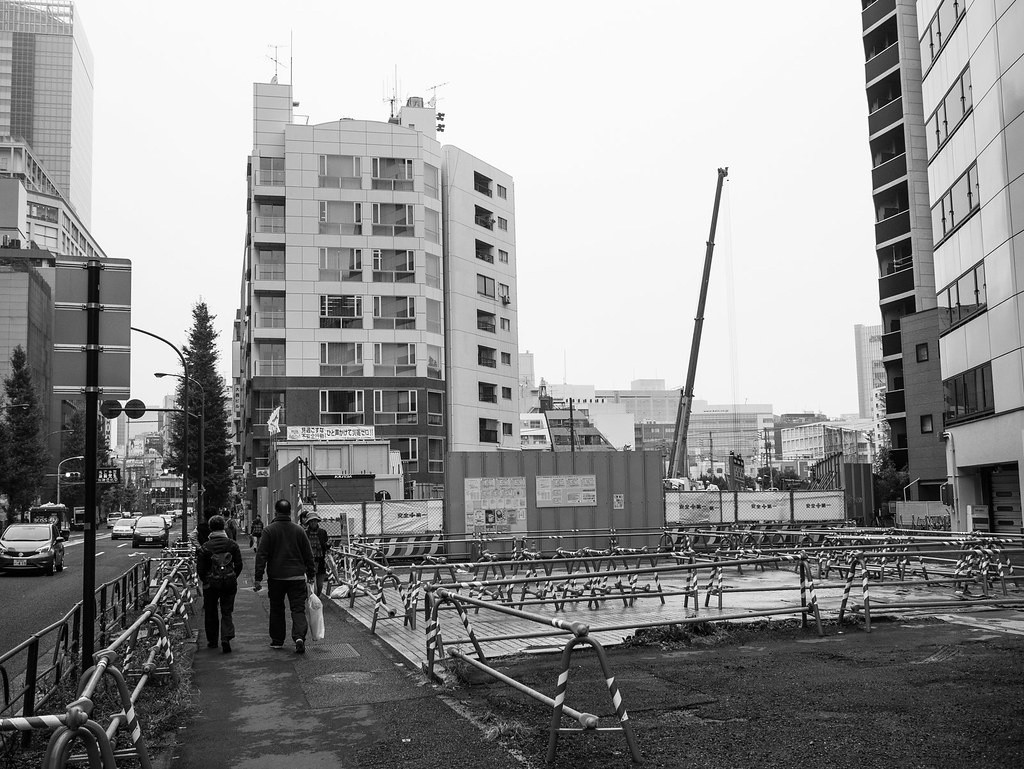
57;456;83;507
154;371;204;525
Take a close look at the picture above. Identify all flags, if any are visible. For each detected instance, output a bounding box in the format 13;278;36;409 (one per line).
267;406;281;437
429;96;438;107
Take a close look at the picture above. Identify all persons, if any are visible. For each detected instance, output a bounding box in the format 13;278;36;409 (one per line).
302;511;335;599
197;514;212;547
222;509;238;543
296;510;311;532
220;504;246;533
194;514;243;653
250;514;265;553
252;498;317;653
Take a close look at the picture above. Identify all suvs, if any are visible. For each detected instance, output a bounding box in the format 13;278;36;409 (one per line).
132;516;172;549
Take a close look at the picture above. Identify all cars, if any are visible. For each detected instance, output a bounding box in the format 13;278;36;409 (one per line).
130;512;144;520
122;511;131;518
173;509;184;519
111;519;137;540
165;511;177;523
187;507;193;517
159;514;175;530
0;523;66;577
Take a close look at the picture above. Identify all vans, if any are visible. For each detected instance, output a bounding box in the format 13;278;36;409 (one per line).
106;511;123;530
30;504;70;542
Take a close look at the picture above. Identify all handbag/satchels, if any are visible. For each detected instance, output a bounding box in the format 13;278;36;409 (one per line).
305;582;326;642
249;537;253;549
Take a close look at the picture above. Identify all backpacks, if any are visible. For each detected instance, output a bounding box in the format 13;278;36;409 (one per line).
224;518;233;540
201;538;237;596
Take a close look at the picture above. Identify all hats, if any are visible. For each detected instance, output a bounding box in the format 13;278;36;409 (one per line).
304;511;322;525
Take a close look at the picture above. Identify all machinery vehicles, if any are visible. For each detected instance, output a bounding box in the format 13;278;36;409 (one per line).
663;165;728;491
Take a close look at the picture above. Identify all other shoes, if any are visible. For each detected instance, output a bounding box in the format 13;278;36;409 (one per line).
254;547;257;553
221;641;232;654
269;640;285;649
295;638;305;654
206;641;217;648
